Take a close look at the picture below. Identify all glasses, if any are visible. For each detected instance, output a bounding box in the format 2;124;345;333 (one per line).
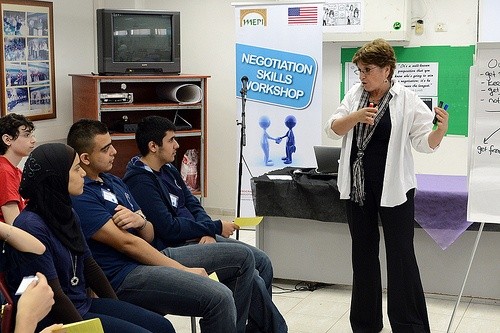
354;66;379;75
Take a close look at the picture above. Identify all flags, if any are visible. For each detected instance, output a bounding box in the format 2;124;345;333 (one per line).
287;7;319;25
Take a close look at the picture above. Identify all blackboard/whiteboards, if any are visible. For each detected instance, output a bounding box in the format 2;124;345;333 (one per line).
467;41;500;225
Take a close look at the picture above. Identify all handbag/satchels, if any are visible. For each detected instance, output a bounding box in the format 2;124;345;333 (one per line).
181;149;199;192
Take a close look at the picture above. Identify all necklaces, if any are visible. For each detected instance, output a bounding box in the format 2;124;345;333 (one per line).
67;247;79;286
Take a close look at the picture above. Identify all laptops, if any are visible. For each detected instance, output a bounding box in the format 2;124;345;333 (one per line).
314;146;342;175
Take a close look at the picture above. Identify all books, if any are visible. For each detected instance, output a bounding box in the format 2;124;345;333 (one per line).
53;317;104;333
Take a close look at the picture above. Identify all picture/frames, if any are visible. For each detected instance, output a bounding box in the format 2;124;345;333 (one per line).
0;0;57;121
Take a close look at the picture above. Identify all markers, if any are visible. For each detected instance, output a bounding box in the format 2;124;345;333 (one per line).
433;101;444;124
438;104;448;122
368;102;374;117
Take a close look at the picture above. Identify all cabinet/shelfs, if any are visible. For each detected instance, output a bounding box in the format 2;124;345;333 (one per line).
68;72;210;208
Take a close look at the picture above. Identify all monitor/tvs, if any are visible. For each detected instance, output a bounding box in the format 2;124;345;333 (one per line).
96;9;180;76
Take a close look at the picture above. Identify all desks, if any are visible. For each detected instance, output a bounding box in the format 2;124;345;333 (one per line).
250;167;500;302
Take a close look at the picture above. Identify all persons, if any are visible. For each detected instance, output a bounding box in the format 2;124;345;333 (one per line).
4;15;51;111
67;118;255;333
0;222;64;333
120;116;290;333
323;37;448;333
0;113;37;231
3;143;175;333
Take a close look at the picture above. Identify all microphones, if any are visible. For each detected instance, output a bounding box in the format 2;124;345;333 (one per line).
241;76;248;100
